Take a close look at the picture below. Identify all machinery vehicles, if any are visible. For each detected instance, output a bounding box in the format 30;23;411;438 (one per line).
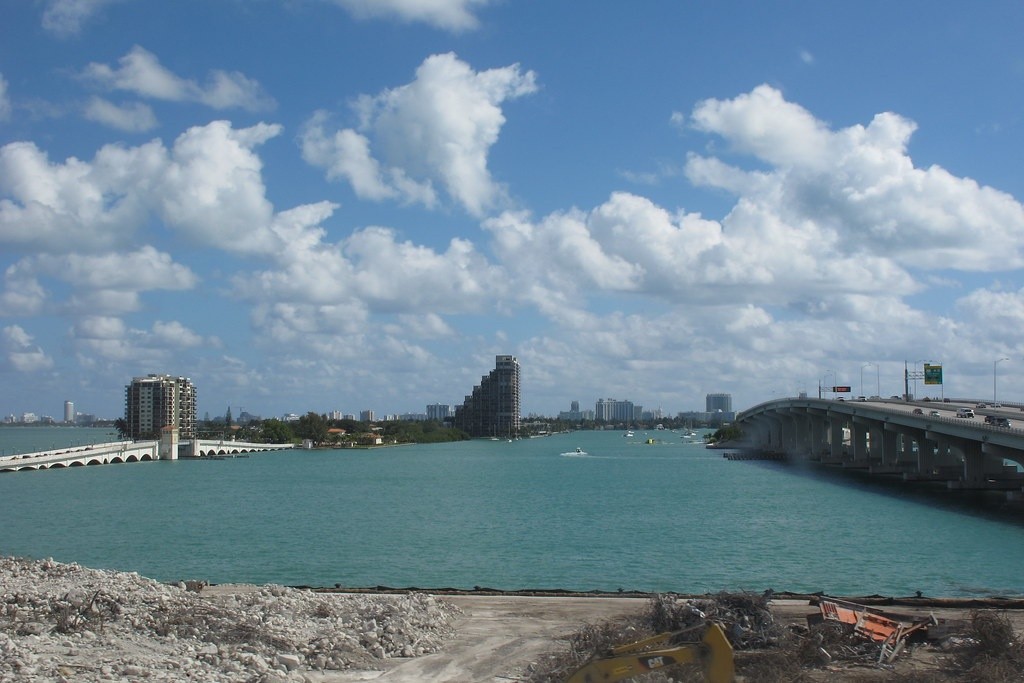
567;619;736;683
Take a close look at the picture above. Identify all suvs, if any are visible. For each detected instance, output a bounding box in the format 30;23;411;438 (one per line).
957;408;975;419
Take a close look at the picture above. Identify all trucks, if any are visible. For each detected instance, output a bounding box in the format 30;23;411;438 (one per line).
902;394;915;400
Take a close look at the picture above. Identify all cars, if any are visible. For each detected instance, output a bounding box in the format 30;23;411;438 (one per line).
975;402;986;409
923;397;931;402
943;397;950;402
890;395;900;399
837;395;845;402
858;395;868;402
985;413;1012;428
913;408;924;415
930;410;941;417
990;402;1002;407
869;395;882;400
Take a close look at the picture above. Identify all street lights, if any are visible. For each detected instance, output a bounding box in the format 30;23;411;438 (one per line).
869;361;879;396
823;373;833;398
993;357;1010;409
827;369;836;398
915;359;926;402
929;359;943;402
860;363;872;394
